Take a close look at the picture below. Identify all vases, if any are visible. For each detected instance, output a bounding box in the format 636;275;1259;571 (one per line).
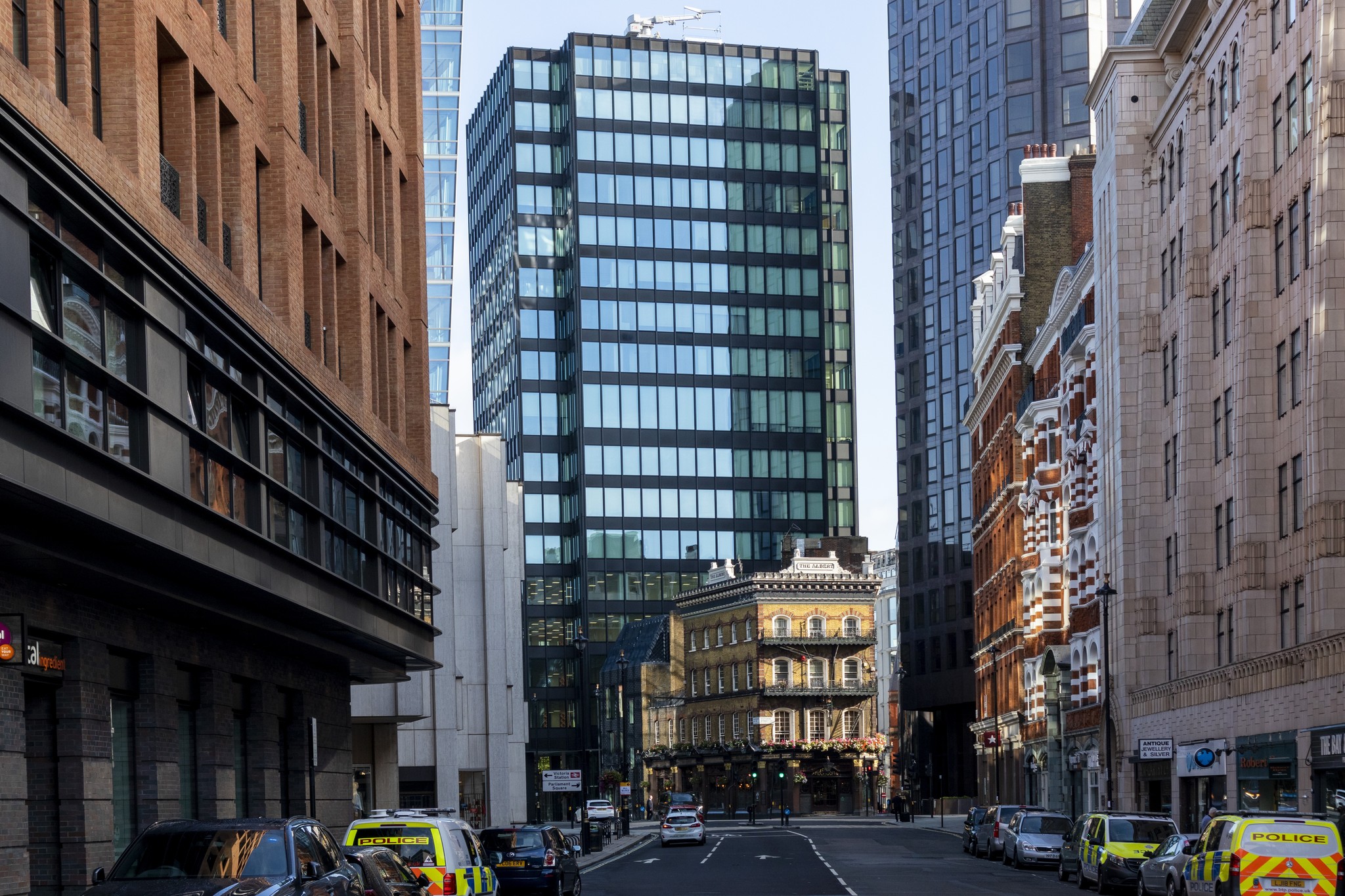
644;785;651;787
693;780;702;784
669;784;674;787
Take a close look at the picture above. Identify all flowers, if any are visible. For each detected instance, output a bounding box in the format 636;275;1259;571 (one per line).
793;771;808;784
597;767;624;794
689;775;702;785
715;777;730;786
648;743;668;753
877;770;890;789
776;680;786;685
854;772;865;786
672;736;749;752
760;732;888;754
664;779;676;789
641;781;651;787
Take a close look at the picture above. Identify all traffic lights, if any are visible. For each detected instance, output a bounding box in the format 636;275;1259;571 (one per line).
867;766;873;776
872;771;879;776
779;759;786;777
750;759;757;778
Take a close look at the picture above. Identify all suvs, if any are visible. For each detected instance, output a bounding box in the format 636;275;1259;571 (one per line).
1002;809;1074;871
657;791;704;815
1058;813;1094;882
478;824;582;896
340;807;503;896
974;805;1047;861
1180;810;1345;896
961;805;991;854
1077;810;1180;896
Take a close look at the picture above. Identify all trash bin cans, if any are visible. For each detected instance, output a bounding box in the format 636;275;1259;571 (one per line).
590;822;603;852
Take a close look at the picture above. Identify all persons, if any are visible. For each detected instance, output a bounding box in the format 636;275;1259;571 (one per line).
891;792;902;822
646;795;654;820
353;782;362;820
1201;808;1217;833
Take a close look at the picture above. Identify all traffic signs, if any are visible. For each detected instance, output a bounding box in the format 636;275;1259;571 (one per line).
543;780;582;791
542;770;581;780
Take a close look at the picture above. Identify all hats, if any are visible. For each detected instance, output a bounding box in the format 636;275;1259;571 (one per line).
896;792;899;795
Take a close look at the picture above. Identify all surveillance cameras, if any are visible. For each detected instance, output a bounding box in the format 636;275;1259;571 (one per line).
1307;756;1312;763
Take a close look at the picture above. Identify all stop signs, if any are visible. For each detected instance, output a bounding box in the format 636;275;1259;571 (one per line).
882;793;887;797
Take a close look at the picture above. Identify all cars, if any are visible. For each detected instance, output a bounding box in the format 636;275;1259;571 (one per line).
574;799;615;823
658;804;705;827
1137;833;1202;896
660;812;707;848
338;845;434;896
76;816;365;896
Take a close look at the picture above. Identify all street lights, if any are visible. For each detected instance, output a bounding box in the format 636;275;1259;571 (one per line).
1095;574;1118;809
985;641;1002;804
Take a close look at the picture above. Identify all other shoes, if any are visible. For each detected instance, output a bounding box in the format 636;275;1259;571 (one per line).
896;821;898;823
900;821;902;822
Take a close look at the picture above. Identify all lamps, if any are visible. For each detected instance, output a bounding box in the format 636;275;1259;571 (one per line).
1302;789;1314;807
